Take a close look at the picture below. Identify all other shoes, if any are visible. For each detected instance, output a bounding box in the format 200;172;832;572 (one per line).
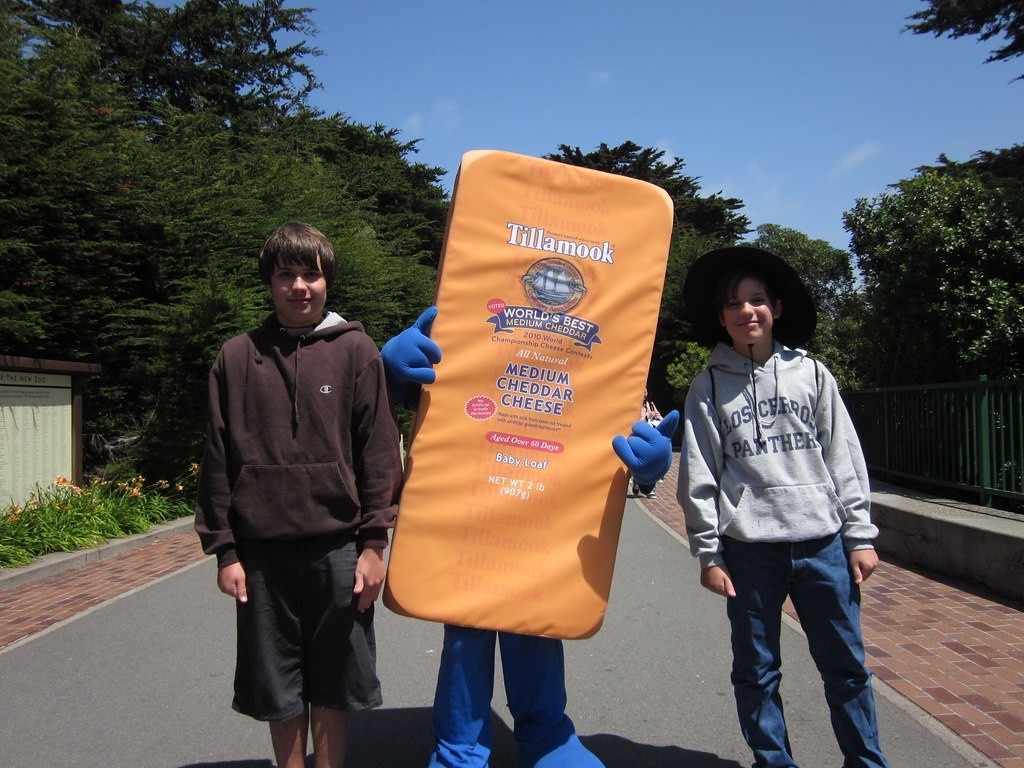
632;479;640;496
647;490;657;499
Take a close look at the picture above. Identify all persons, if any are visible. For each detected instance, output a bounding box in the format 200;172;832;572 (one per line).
632;388;664;499
194;220;404;768
382;304;680;768
676;246;890;768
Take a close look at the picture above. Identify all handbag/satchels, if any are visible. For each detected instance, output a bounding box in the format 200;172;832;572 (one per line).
645;401;661;428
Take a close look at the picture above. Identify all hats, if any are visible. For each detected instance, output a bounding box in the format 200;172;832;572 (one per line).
683;247;817;350
